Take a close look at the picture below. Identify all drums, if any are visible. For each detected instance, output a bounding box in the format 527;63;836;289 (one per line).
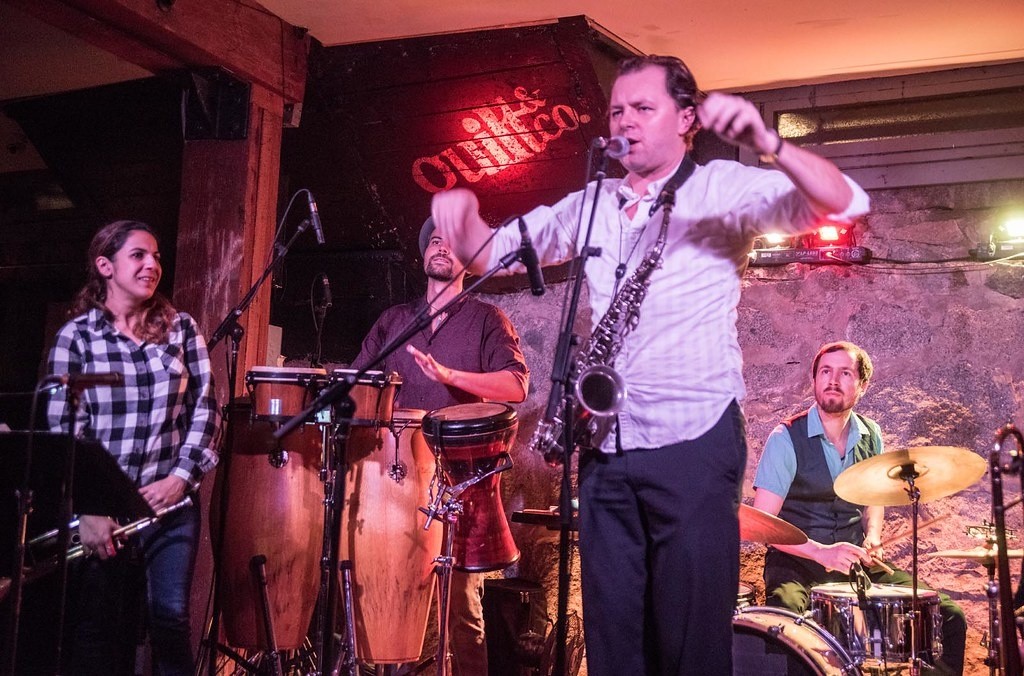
732;606;863;676
244;365;403;432
421;402;522;572
810;581;945;670
208;396;445;664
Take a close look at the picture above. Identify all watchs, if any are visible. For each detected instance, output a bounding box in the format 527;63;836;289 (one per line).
756;127;782;163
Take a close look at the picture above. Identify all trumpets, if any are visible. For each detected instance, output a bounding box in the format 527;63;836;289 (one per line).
0;496;193;598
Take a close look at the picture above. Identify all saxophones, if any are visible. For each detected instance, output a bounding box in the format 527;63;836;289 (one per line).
524;184;677;467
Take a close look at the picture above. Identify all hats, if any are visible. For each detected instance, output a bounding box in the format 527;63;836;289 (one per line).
418;215;437;257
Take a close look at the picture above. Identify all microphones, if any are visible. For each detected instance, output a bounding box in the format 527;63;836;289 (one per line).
593;135;631;158
62;371;125;387
323;274;333;307
519;224;547;296
307;192;326;245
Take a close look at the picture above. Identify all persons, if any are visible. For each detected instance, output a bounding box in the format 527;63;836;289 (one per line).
346;212;529;676
42;221;217;676
431;51;870;675
753;342;966;676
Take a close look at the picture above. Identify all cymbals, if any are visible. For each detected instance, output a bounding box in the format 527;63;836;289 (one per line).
927;542;1024;565
737;503;809;546
833;446;987;507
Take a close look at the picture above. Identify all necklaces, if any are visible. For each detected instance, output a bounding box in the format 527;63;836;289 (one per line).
430;304;445;328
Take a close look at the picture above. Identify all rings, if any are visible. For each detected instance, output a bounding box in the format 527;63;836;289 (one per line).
105;544;114;549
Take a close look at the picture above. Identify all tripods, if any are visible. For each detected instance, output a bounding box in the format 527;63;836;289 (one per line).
199;219;306;676
